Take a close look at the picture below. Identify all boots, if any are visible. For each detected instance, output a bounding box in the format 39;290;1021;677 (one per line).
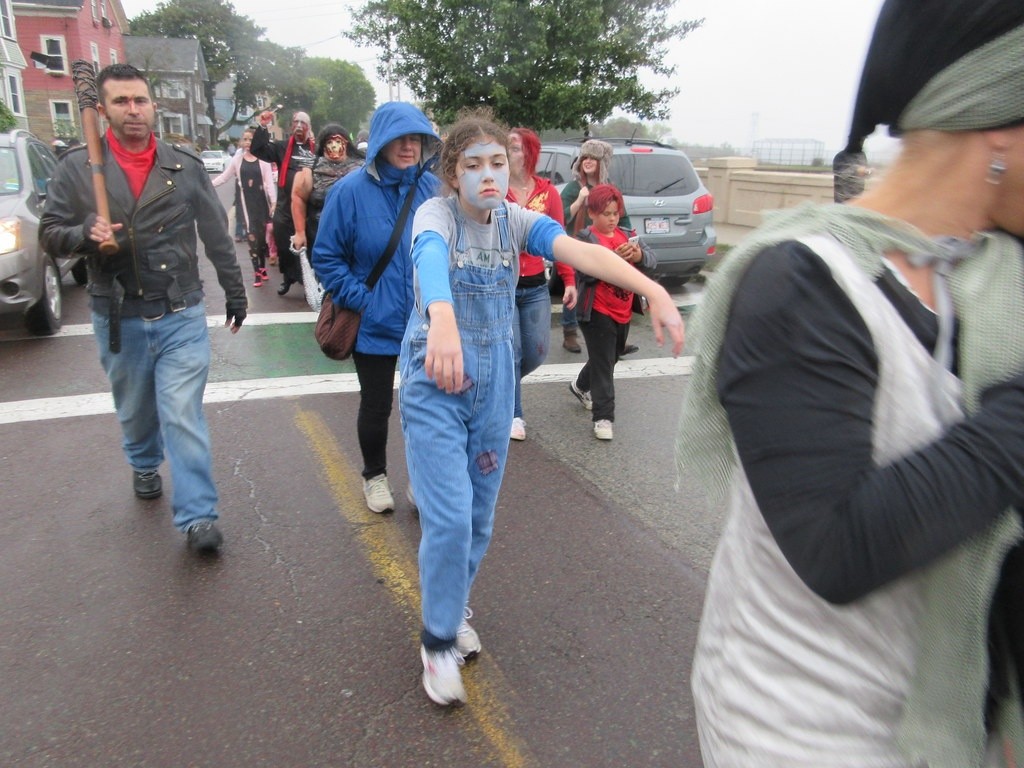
621;344;639;355
563;329;581;352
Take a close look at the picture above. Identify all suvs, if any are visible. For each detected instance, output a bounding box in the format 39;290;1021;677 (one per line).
0;129;88;337
535;136;719;288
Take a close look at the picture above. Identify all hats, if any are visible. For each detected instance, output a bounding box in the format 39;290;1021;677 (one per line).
835;1;1024;201
572;140;613;184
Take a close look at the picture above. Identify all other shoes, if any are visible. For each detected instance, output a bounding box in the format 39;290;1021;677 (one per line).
253;273;262;286
259;268;269;281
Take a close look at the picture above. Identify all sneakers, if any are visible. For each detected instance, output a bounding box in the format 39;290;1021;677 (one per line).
594;419;613;439
510;417;526;440
569;379;593;411
452;605;481;660
188;522;220;551
420;644;465;706
363;473;394;513
133;469;162;497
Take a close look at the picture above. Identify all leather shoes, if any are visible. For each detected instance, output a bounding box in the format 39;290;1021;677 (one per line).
277;275;296;295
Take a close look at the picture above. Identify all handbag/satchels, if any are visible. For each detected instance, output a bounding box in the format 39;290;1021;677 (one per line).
315;290;363;362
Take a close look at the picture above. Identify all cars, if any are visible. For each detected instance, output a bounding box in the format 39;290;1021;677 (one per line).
201;144;237;173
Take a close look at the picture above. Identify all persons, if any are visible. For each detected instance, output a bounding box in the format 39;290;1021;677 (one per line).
505;127;576;442
560;140;657;439
833;135;868;202
290;124;366;252
692;0;1024;768
312;101;449;512
211;127;277;287
38;64;249;555
398;121;686;708
250;110;314;295
